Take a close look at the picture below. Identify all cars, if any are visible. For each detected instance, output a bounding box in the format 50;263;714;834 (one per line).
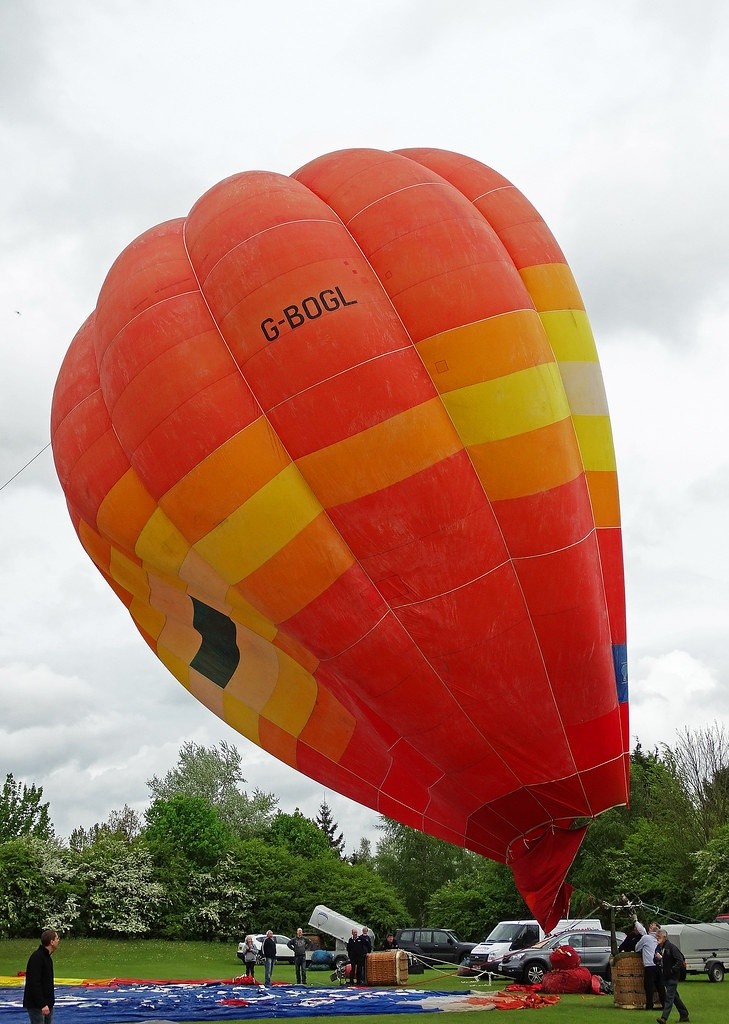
497;929;629;984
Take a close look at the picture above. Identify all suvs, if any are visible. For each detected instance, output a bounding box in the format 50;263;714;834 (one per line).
393;926;478;968
237;934;296;965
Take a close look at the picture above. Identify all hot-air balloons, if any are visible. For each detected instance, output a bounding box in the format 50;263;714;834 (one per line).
46;147;647;1010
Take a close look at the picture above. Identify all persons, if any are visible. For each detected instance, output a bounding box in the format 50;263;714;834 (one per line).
287;927;314;984
261;930;276;988
23;930;60;1024
243;936;259;978
381;933;399;950
346;927;371;985
618;923;643;952
632;914;666;1011
653;929;690;1024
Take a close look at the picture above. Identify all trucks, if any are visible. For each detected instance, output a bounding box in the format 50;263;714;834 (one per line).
300;905;374;971
469;919;602;979
660;922;729;983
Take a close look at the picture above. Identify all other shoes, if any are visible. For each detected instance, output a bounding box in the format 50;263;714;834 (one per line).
657;1018;666;1024
264;984;270;987
679;1017;690;1022
